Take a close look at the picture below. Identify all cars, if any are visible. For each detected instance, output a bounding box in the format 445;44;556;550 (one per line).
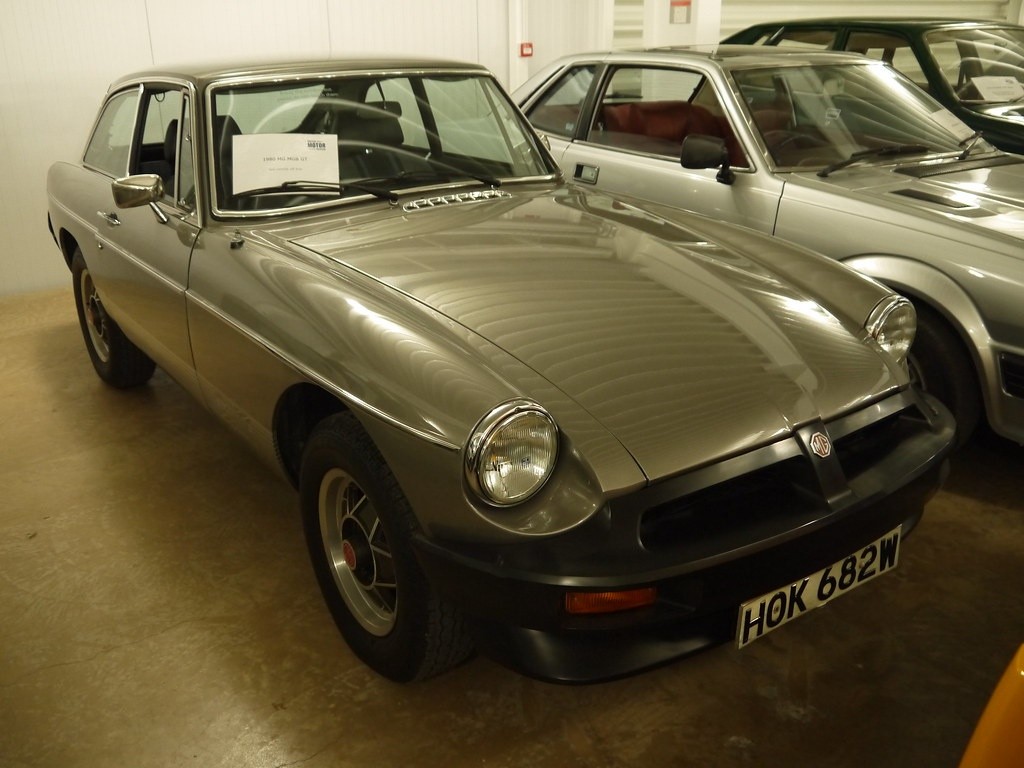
717;12;1024;157
43;50;963;697
507;37;1024;454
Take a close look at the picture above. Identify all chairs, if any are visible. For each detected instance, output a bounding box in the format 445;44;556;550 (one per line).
731;108;805;168
164;115;243;212
328;106;437;196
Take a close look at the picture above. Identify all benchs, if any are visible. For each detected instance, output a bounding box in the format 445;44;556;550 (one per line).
533;100;729;159
